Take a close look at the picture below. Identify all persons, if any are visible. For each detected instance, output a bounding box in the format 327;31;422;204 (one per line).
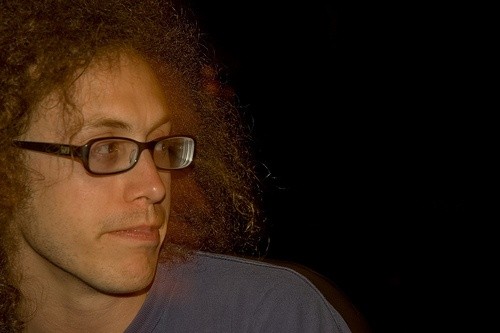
0;0;353;332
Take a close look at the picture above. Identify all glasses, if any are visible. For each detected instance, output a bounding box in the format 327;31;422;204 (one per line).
9;136;196;176
